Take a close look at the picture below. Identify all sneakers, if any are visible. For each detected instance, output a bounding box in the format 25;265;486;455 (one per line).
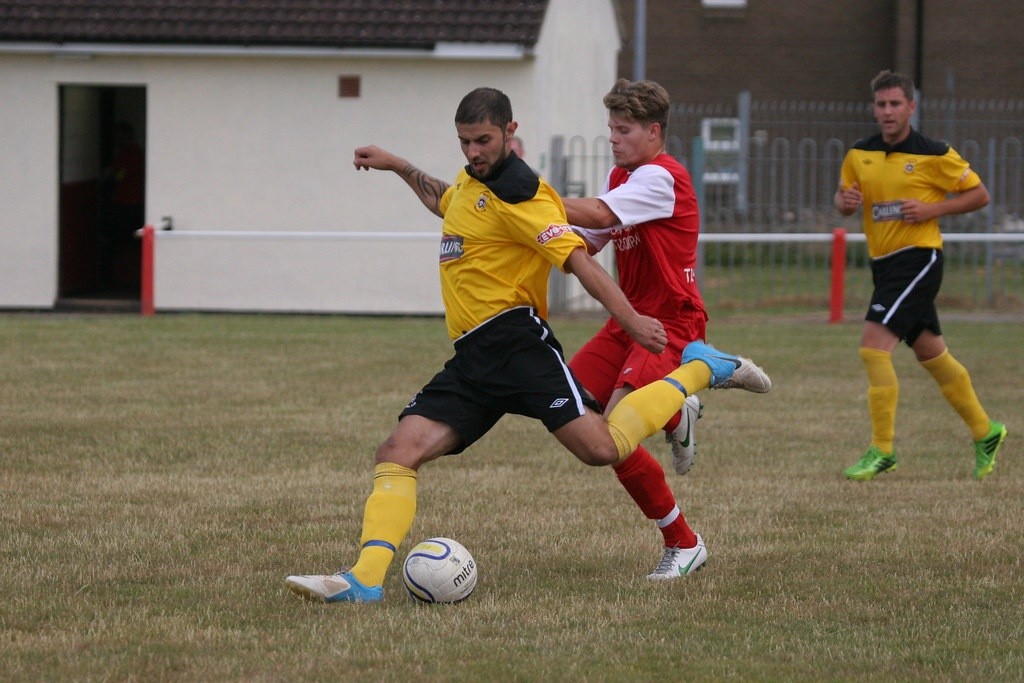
285;569;384;602
666;393;703;476
844;444;897;481
972;420;1007;480
650;532;707;581
679;339;772;394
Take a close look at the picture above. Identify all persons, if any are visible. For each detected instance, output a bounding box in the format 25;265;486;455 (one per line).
284;88;772;603
834;73;1008;479
559;77;708;579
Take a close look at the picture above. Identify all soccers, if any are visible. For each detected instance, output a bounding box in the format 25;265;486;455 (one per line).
403;537;480;606
